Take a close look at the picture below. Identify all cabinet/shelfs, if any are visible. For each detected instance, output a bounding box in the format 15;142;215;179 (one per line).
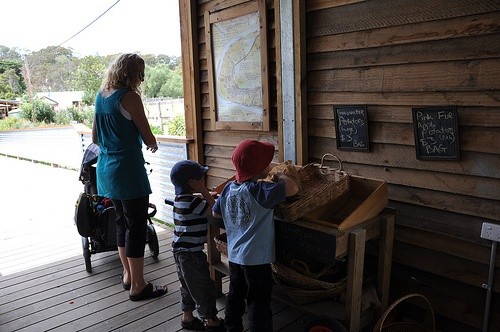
206;199;395;332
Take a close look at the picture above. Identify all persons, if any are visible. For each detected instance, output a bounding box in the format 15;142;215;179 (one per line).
169;159;227;332
211;139;299;332
92;52;169;301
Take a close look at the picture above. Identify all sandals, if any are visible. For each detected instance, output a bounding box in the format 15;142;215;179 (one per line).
181;316;203;330
205;317;226;331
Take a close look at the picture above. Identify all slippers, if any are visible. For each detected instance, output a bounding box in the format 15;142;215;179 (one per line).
129;282;168;301
120;271;131;290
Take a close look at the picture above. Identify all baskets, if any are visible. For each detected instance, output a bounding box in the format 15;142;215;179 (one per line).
272;154;351;222
370;294;436;332
271;273;344;304
214;232;228;257
271;260;348;290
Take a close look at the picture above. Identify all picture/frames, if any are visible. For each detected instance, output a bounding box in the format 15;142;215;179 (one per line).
412;106;462;162
333;104;370;152
204;0;270;132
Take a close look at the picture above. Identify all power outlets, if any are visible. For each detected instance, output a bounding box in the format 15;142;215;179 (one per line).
480;221;500;242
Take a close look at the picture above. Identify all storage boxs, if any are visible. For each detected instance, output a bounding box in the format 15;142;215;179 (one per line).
266;163;390;229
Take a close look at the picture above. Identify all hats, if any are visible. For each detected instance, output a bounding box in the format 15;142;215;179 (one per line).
232;140;275;181
170;160;208;195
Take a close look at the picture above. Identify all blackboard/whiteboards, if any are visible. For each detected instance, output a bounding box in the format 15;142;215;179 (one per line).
412;108;461;161
333;105;370;153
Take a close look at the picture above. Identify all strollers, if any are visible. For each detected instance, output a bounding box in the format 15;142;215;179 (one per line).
74;132;159;273
